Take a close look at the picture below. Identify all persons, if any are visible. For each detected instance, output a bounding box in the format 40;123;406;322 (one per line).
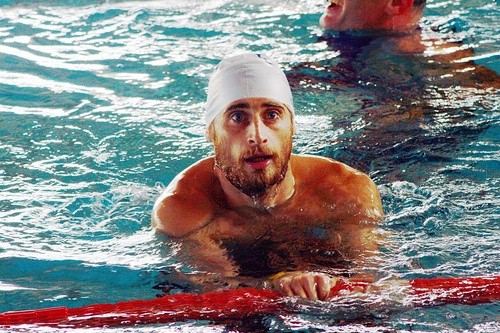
151;52;394;299
284;0;500;185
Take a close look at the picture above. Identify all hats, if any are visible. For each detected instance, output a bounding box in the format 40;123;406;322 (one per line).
205;51;294;130
